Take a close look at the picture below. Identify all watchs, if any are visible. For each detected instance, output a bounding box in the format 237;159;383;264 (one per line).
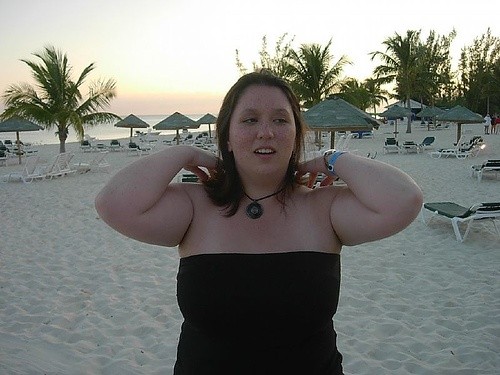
324;149;337;176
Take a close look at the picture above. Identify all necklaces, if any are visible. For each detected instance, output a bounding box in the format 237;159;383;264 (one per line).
243;187;284;219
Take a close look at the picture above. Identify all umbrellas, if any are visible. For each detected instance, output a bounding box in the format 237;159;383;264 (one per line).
378;105;415;132
114;114;149;137
153;112;200;145
416;106;446;131
437;105;486;143
196;113;218;138
301;94;380;149
0;114;44;164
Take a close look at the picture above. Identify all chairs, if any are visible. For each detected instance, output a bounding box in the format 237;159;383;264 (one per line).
0;119;500;243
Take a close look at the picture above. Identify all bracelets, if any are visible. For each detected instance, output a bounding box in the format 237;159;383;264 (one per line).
327;150;349;177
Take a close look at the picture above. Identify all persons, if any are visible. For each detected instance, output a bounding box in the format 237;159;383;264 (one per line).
484;113;491;134
491;117;496;134
94;72;424;375
496;116;500;134
